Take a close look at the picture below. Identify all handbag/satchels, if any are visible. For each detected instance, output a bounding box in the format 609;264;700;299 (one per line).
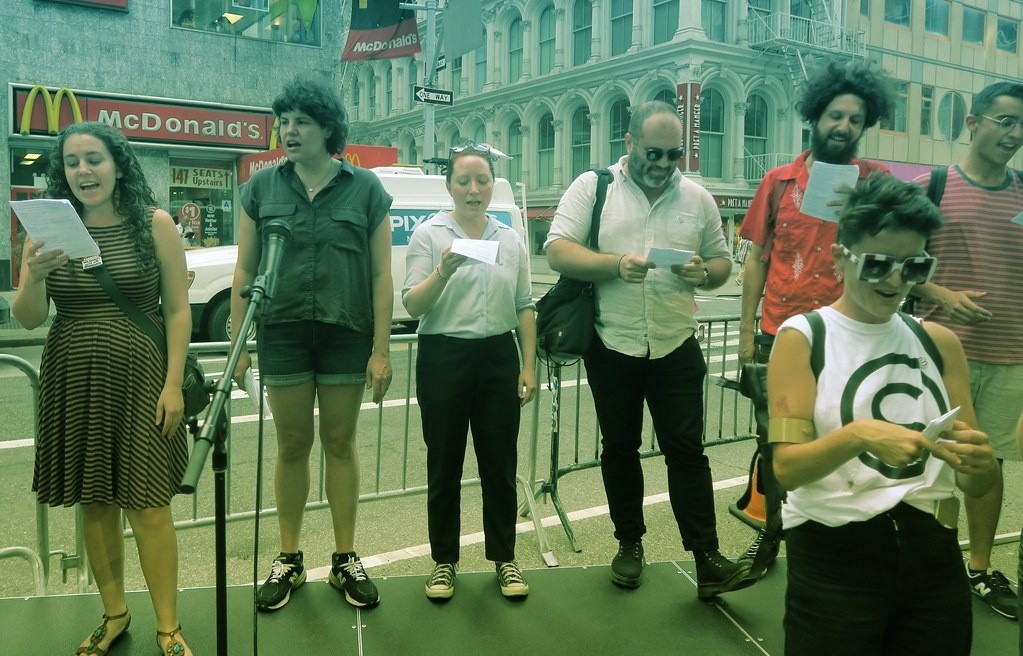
535;275;594;362
181;353;210;416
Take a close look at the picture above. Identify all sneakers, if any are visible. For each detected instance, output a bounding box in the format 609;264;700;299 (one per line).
737;527;780;577
257;550;307;610
328;552;380;607
426;563;456;599
965;560;1017;618
496;561;529;596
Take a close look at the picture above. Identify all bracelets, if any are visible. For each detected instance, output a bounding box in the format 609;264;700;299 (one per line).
436;265;447;282
698;268;708;287
618;255;626;277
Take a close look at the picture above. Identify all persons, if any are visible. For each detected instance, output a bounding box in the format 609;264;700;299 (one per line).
176;215;195;246
739;58;897;565
766;171;999;656
229;84;393;610
906;81;1023;620
11;123;193;655
544;100;750;602
400;146;539;599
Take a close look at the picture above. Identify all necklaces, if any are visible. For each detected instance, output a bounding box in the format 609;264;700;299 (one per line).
309;160;332;192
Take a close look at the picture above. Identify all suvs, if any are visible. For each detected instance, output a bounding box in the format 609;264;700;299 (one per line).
160;166;526;359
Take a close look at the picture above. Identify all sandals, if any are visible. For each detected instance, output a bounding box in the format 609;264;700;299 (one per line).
156;626;193;656
76;605;131;656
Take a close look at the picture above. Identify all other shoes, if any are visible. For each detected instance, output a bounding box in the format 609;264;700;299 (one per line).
610;537;645;587
693;549;758;598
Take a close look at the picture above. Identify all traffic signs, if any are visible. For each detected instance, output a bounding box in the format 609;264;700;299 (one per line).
413;85;454;107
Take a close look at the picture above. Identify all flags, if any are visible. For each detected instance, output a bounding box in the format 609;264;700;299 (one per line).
341;0;422;63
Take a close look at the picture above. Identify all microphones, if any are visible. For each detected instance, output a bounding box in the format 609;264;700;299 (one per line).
260;220;290;315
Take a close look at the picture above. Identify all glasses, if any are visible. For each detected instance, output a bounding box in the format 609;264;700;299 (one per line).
975;113;1023;131
629;132;683;161
839;243;937;285
448;144;491;169
